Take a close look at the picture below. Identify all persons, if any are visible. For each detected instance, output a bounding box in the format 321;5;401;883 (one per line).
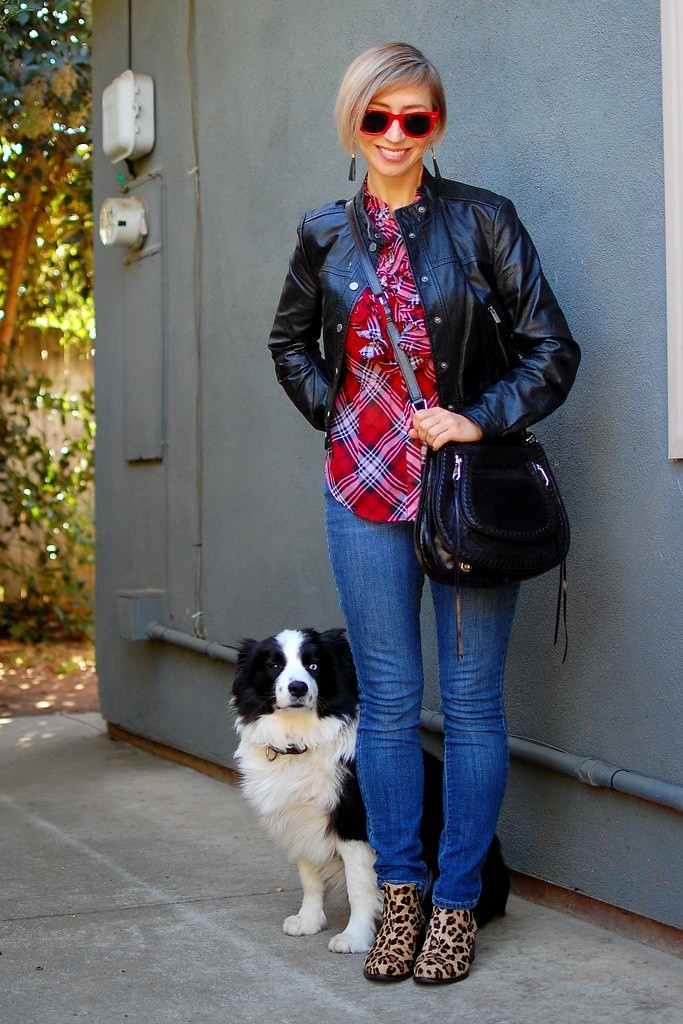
267;42;582;986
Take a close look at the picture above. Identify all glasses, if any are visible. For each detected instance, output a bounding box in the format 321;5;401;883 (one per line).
355;108;442;139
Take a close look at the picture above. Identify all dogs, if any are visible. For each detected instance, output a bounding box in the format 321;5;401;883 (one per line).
225;627;510;954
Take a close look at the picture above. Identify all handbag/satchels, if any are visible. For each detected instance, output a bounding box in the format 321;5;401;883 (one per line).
410;429;571;587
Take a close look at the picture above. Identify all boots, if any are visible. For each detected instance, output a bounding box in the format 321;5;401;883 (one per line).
414;903;478;987
364;881;424;980
